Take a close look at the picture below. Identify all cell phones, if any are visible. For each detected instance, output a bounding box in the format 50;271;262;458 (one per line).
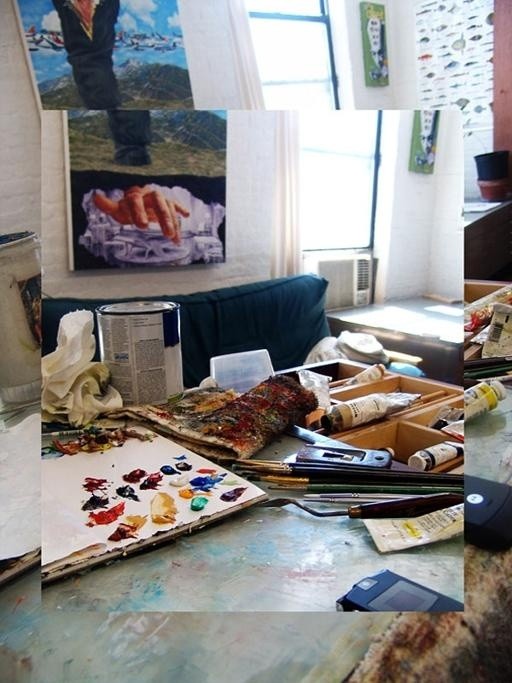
464;474;512;552
336;570;464;611
296;444;392;471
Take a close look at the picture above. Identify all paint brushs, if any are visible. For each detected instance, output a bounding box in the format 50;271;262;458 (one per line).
464;356;512;382
217;456;464;503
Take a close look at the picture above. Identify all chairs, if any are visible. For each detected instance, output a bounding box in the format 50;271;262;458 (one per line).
45;275;326;411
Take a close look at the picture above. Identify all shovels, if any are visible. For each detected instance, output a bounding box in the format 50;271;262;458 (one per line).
254;493;464;518
